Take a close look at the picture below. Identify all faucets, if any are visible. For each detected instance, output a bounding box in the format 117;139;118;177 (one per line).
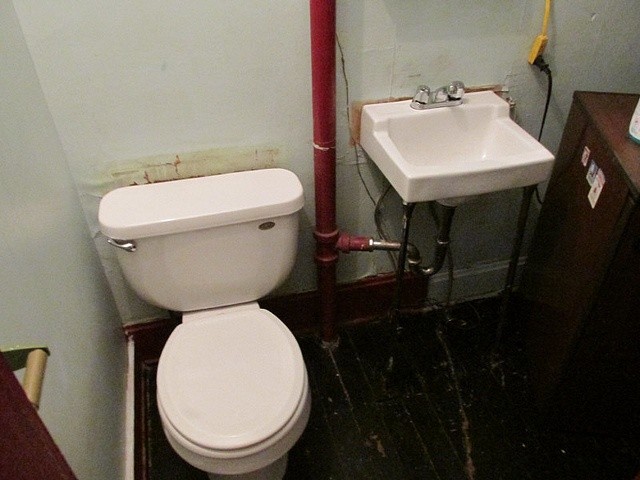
429;85;457;105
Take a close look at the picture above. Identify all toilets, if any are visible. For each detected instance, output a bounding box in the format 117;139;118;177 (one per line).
97;167;310;480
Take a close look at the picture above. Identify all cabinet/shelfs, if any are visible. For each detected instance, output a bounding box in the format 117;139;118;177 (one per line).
509;89;640;384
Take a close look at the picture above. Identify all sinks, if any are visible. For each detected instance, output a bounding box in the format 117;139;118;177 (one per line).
359;90;556;203
